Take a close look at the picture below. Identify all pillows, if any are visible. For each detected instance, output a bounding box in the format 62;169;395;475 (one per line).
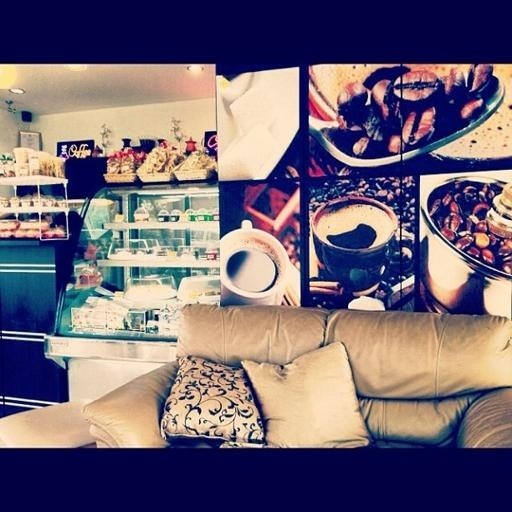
160;354;265;444
241;342;370;449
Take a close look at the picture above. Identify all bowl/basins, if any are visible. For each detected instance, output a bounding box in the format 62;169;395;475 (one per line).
421;173;512;316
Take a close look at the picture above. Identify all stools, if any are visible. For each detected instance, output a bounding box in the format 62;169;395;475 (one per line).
0;402;95;448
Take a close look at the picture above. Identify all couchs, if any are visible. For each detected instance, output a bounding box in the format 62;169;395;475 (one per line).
85;304;511;450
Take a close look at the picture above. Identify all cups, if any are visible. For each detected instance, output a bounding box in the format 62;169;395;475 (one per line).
220;220;290;307
307;70;505;168
311;196;414;292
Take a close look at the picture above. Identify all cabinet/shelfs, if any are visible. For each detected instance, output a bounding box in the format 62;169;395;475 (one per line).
72;220;220;332
0;175;68;240
